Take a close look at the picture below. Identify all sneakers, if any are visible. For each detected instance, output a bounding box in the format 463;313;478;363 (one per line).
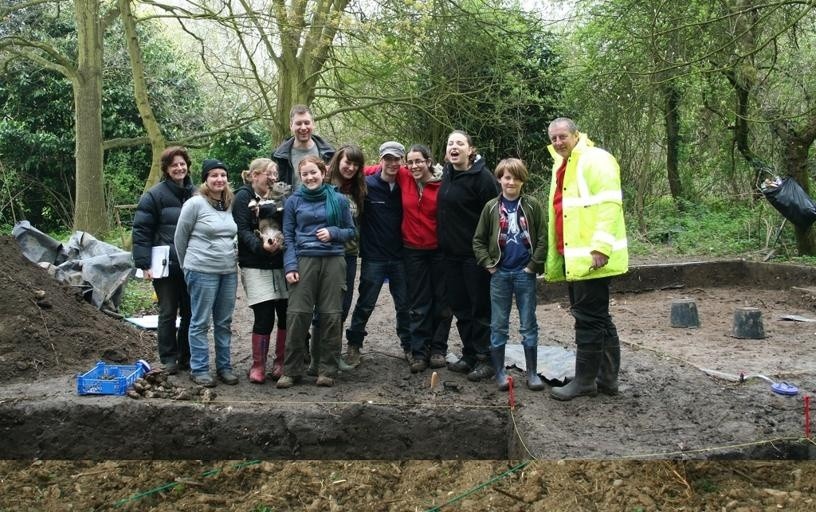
402;350;415;364
467;360;495;381
448;357;472;372
163;362;178;373
410;354;430;373
315;373;336;386
188;371;217;387
275;374;298;388
341;344;363;370
429;354;446;368
217;369;239;385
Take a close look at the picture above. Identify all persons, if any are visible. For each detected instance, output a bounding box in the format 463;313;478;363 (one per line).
544;116;629;401
307;142;368;379
232;159;287;383
272;104;336;364
435;130;501;383
174;161;239;386
366;144;454;372
344;141;416;367
473;158;549;390
131;147;198;375
276;154;355;389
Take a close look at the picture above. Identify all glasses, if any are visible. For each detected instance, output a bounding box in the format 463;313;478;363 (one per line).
262;171;278;177
408;159;427;165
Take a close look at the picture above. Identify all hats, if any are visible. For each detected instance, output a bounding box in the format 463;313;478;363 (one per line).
379;141;405;159
201;158;228;182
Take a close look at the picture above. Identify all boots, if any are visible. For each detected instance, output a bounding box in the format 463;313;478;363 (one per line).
595;344;621;395
271;328;287;380
490;344;514;391
248;332;270;384
523;345;544;391
551;348;604;400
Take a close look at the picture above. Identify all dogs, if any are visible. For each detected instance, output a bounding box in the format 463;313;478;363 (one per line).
254;176;293;252
